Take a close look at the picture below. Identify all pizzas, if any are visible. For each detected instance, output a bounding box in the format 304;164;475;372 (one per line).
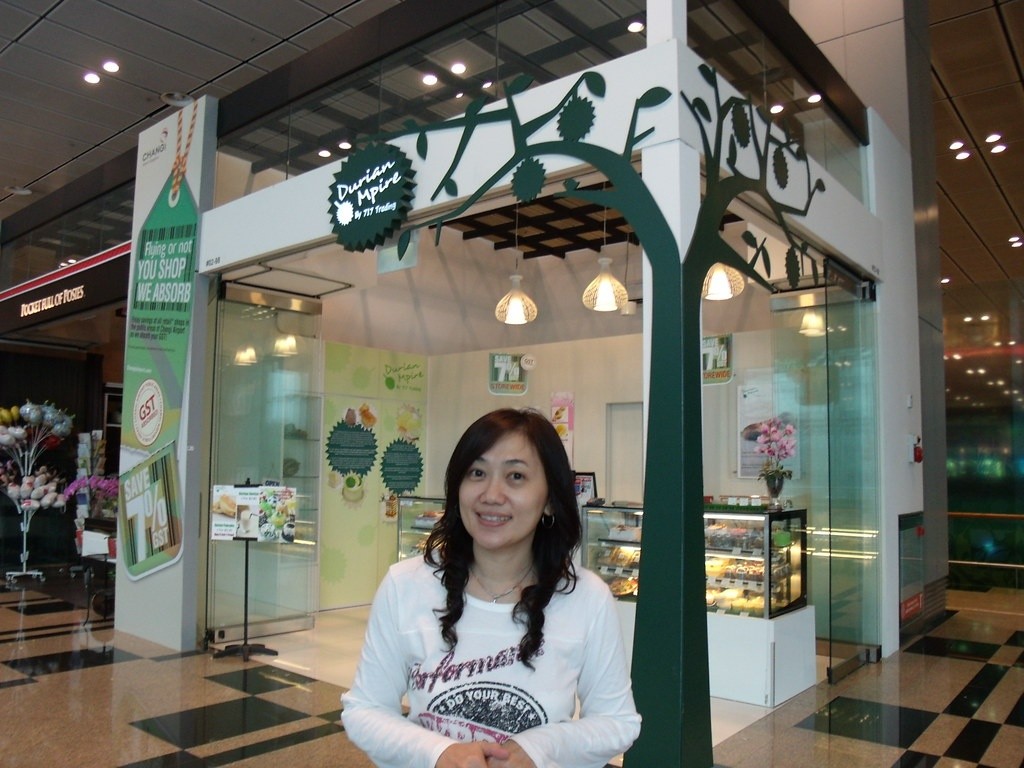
608;579;637;596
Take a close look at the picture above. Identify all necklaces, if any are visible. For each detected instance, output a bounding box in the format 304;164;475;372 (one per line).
471;563;532;604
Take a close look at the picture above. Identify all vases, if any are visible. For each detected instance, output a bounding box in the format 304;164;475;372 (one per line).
766;474;784;498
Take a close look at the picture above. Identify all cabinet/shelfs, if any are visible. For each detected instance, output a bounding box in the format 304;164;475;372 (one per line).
599;519;791;614
409;509;445;555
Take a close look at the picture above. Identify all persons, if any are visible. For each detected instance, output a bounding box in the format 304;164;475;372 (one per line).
340;409;643;768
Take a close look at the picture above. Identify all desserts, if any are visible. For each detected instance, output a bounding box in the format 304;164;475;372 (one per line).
704;527;791;615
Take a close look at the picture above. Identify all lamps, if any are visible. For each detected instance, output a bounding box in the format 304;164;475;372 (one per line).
582;182;629;312
701;262;745;300
495;204;538;324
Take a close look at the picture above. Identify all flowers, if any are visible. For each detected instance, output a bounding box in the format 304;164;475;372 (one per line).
753;417;798;482
